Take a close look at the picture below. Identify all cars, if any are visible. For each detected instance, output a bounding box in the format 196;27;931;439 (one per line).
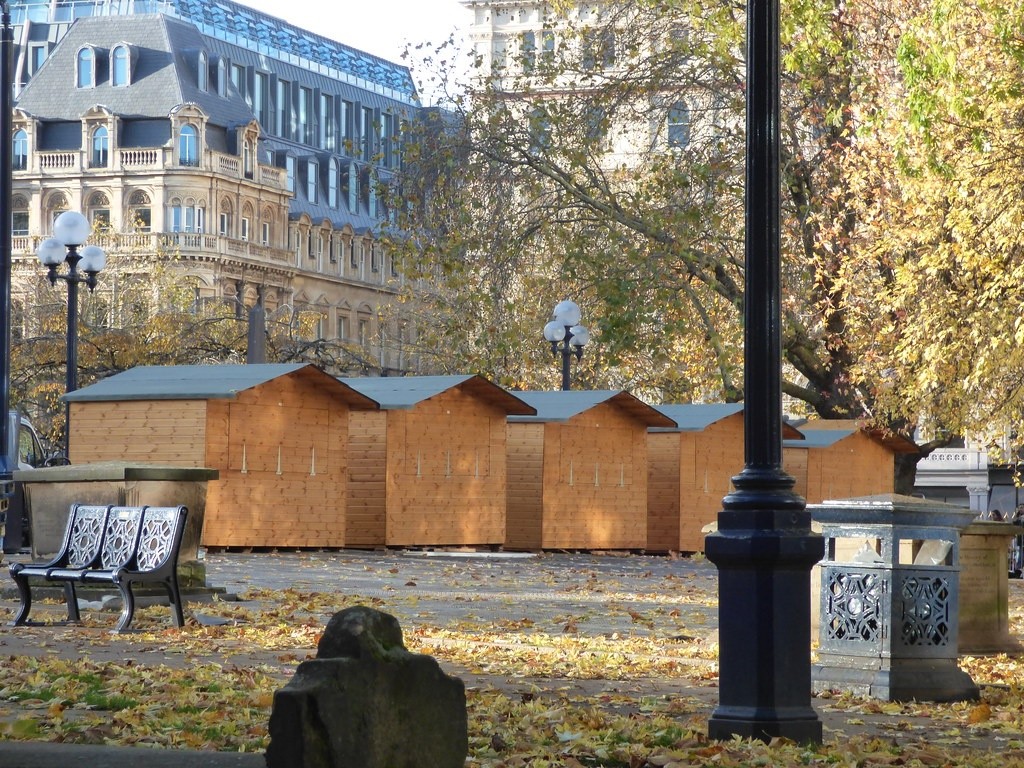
18;417;71;470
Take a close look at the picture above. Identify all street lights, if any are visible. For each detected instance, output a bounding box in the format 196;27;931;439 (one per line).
37;211;106;459
544;301;590;391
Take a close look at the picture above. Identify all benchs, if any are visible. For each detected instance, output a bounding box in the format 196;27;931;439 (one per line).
9;503;188;635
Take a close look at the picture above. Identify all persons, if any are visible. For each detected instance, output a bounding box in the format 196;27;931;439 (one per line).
1013;503;1024;568
992;510;1003;521
